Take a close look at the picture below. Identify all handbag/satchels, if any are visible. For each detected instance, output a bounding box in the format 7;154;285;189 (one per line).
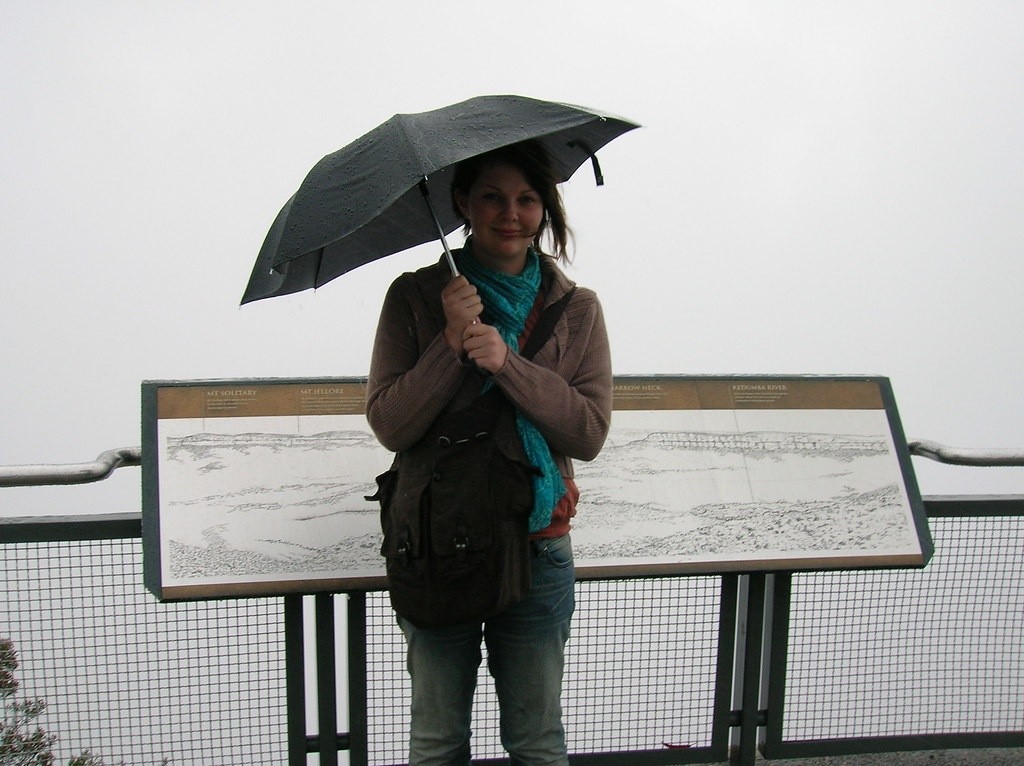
364;412;532;634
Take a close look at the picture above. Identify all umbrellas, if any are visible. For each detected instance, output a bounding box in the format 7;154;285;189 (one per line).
240;94;644;323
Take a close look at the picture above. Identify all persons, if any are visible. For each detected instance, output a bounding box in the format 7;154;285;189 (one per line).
364;137;615;766
